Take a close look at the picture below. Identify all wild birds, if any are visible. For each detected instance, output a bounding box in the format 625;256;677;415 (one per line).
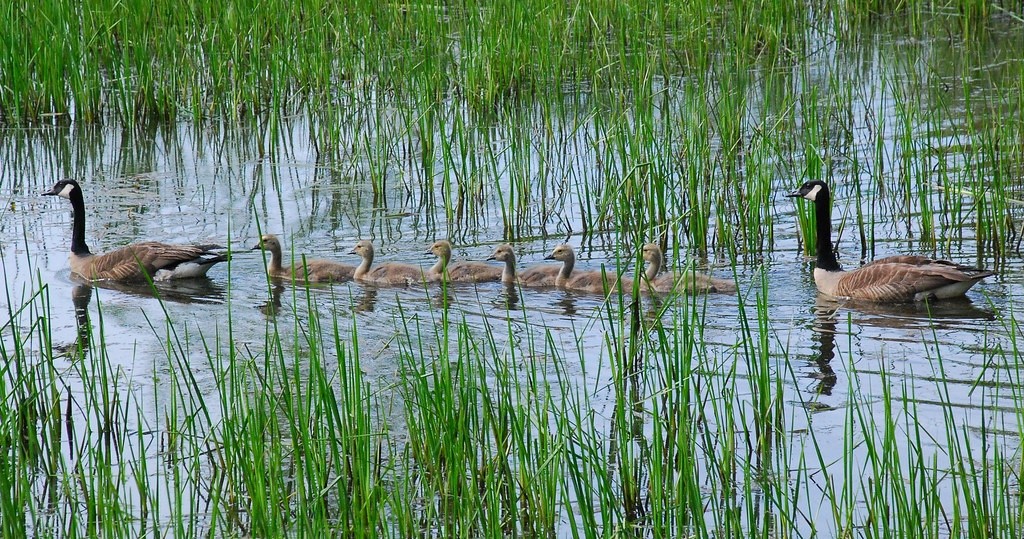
252;234;738;295
41;178;233;282
785;180;997;304
54;280;226;359
